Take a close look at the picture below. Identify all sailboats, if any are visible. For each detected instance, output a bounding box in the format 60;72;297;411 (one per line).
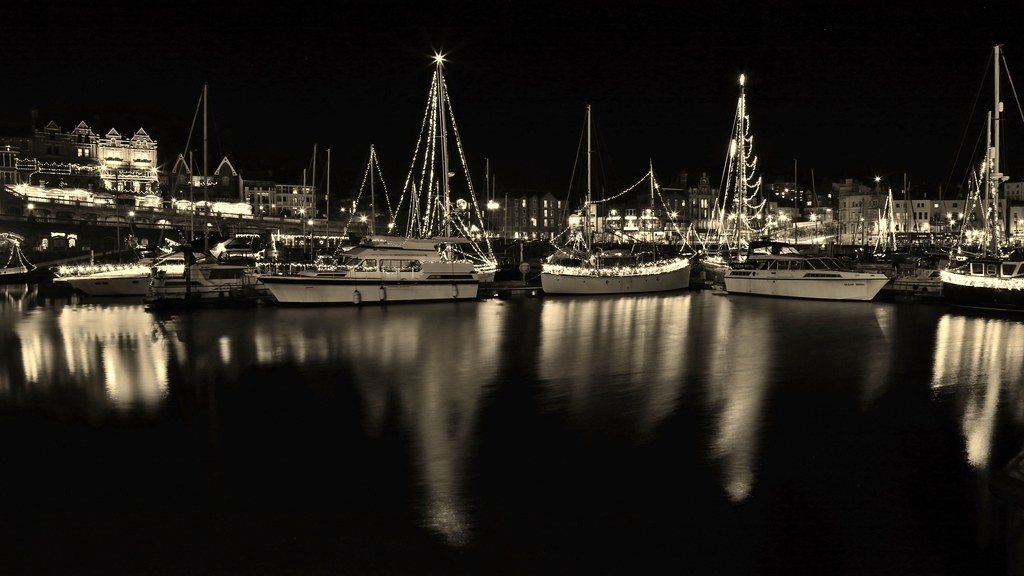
700;73;1024;306
540;102;698;297
47;47;502;313
939;43;1024;318
